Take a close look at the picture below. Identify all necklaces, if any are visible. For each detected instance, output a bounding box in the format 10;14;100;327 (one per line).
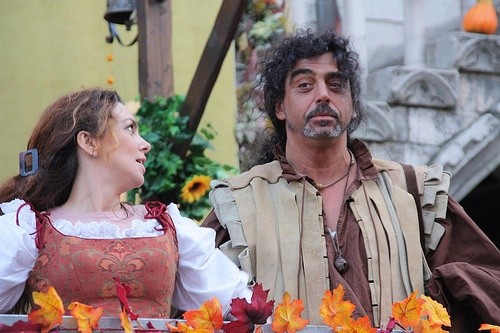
282;148;356;273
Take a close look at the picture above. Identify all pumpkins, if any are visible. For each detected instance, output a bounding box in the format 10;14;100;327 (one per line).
464;0;498;34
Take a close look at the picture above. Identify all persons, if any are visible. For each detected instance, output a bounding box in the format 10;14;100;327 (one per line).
0;86;272;333
196;28;500;333
